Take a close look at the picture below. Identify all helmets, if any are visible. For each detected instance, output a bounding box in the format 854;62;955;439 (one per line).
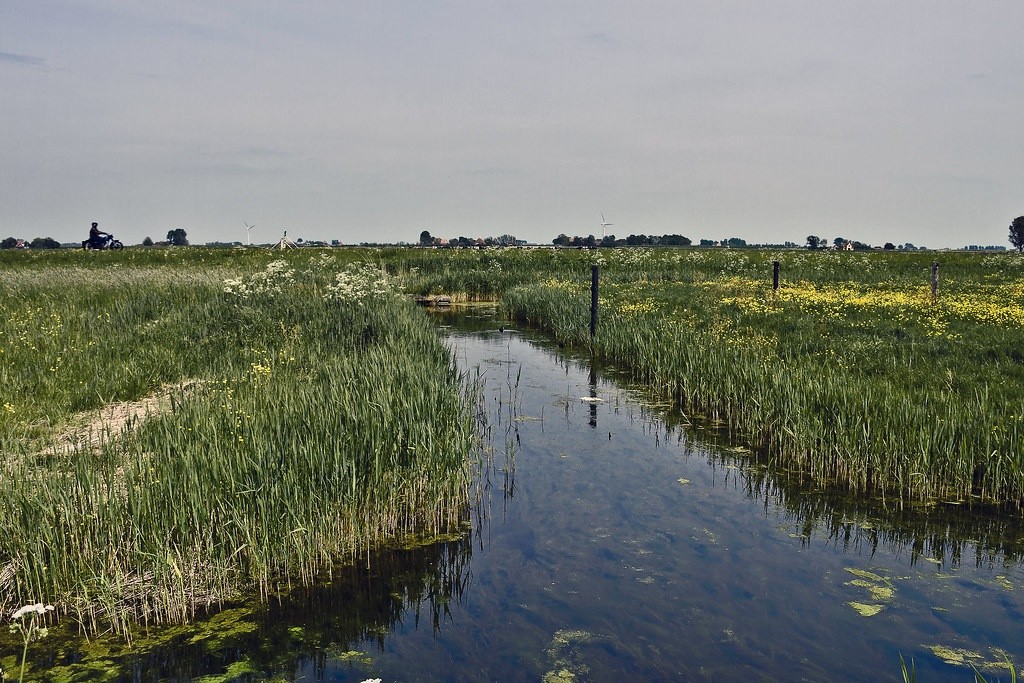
92;222;98;227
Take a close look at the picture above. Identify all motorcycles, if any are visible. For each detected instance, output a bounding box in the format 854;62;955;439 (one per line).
82;234;123;251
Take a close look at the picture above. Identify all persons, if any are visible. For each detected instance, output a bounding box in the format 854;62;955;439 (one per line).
89;222;109;251
